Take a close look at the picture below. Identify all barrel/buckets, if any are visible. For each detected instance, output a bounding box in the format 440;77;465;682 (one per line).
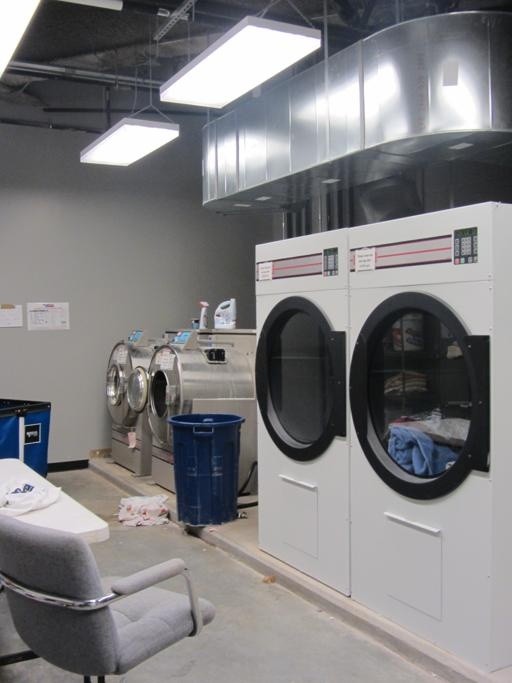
166;413;245;524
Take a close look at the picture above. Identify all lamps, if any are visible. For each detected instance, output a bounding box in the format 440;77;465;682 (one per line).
159;15;322;110
80;40;180;167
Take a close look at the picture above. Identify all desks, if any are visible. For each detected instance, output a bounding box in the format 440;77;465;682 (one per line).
389;416;471;448
0;458;109;546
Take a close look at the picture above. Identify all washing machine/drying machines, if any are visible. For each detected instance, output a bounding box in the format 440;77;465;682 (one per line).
350;201;512;672
254;227;350;596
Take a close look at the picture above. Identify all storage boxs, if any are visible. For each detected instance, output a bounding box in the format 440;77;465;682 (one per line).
1;398;50;479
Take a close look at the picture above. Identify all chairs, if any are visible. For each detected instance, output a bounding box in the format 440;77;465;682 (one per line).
1;515;216;683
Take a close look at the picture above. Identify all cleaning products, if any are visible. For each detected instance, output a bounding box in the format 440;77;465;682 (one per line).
199;301;209;329
213;298;237;329
